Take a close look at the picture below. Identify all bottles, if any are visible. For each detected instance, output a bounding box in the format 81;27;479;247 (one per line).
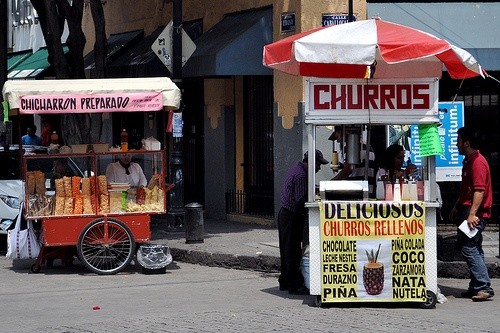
376;167;423;201
121;129;128;151
51;131;58;145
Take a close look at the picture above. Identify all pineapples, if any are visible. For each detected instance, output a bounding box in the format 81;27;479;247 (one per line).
363;243;384;295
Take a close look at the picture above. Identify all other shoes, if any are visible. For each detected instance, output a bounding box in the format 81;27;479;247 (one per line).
279;285;311;295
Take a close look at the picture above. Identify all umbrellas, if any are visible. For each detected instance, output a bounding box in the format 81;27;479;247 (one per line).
263;17;488;181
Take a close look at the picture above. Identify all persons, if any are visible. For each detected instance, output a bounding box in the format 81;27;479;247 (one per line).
376;144;416;201
106;153;148;187
49;158;80;188
450;127;495;300
21;124;43;146
317;124;375;200
277;148;329;295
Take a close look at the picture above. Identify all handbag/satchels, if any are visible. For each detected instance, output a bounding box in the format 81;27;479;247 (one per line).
5;202;41;260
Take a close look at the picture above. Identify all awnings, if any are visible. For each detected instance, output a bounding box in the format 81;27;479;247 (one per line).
366;2;500;70
182;5;273;78
119;19;202;66
7;52;31;71
7;43;69;78
83;29;143;70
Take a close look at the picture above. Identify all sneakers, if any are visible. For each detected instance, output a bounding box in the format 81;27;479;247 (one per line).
461;289;495;301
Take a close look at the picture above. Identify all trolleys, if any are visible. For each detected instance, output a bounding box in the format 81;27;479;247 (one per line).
303;77;443;310
22;149;176;278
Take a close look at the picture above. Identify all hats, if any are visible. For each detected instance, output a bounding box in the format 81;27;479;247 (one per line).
305;149;329;165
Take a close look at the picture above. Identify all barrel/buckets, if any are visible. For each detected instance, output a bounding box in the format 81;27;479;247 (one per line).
301;256;310;289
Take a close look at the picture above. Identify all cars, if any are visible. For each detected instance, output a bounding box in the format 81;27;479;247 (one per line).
0;145;94;236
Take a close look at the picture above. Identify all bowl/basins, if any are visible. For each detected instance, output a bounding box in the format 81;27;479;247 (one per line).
70;144;88;153
92;144;109;152
